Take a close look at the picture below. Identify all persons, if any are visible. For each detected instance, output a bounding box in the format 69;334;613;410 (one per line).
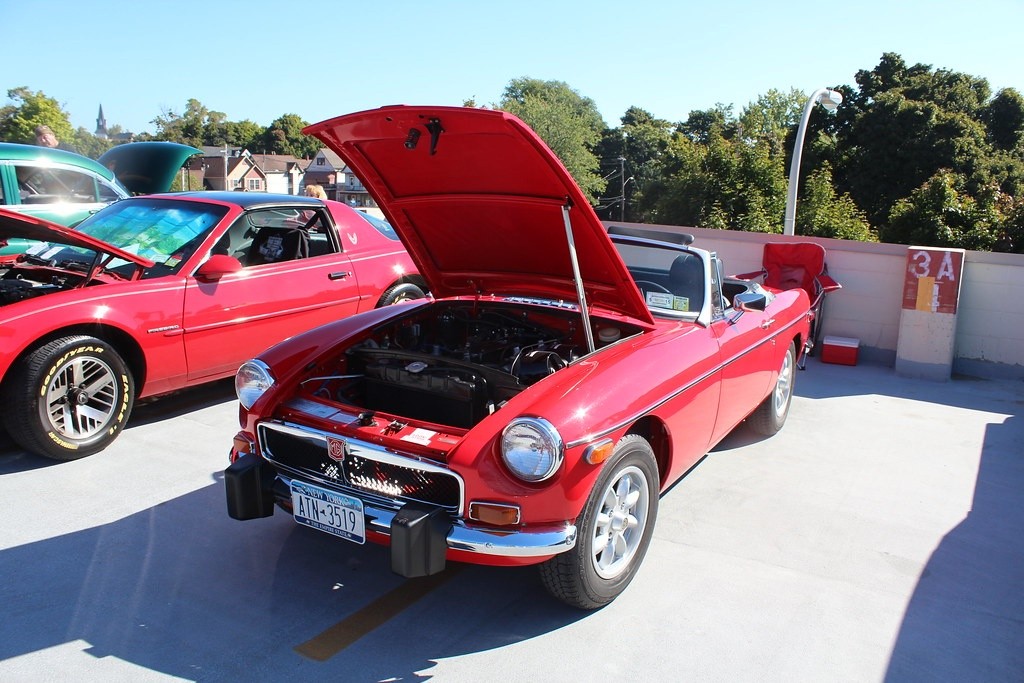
35;125;80;194
283;185;327;228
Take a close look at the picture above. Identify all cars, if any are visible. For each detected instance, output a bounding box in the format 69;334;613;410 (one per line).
0;192;506;461
0;142;204;254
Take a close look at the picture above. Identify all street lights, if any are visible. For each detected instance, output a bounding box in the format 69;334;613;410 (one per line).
783;88;842;236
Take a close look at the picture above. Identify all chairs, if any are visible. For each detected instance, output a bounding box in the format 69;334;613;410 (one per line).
725;241;842;370
211;230;231;256
667;254;733;309
244;228;308;267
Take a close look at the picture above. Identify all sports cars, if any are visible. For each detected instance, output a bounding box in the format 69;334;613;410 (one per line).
225;104;814;610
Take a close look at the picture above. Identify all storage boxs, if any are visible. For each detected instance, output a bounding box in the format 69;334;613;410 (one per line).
822;335;859;366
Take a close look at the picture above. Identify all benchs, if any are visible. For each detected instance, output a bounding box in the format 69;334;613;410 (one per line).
234;235;329;265
